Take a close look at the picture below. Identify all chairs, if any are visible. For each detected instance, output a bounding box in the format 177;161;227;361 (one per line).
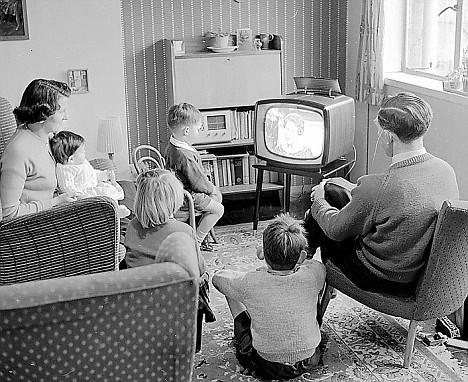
129;141;218;249
313;199;468;368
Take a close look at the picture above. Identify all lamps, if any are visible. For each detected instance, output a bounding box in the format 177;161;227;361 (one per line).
93;115;126;163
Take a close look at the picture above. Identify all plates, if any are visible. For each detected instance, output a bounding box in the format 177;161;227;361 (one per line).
206;46;239;53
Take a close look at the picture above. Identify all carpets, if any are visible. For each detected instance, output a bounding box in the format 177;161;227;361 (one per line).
192;217;468;382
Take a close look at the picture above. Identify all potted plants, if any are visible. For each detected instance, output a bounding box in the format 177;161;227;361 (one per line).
447;69;461;90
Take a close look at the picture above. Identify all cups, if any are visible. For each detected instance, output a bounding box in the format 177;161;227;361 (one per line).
259;33;273;50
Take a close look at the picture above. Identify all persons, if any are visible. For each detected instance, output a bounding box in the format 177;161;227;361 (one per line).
163;103;226;249
0;78;73;219
123;168;215;322
301;92;461;298
47;131;131;220
212;212;329;379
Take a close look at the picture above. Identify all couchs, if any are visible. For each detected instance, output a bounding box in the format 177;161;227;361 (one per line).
0;96;200;381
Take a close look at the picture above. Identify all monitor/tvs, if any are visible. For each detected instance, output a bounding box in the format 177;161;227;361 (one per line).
253;94;355;175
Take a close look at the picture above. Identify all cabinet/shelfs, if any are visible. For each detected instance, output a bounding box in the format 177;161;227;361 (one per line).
164;35;286;217
250;160;355;231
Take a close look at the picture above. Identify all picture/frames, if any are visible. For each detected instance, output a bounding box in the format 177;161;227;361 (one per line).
68;68;89;95
0;1;30;41
